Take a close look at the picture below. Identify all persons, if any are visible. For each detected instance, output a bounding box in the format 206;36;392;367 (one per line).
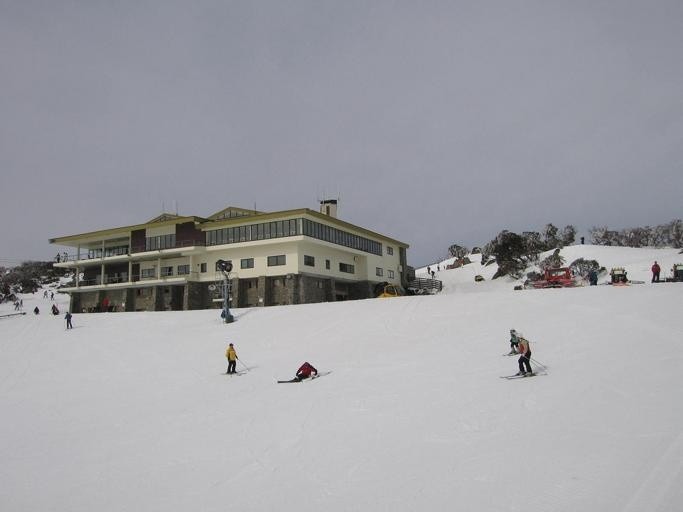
225;343;238;373
34;307;39;314
516;331;531;376
64;312;72;329
652;260;660;283
52;304;59;315
510;330;518;354
44;290;48;298
13;300;19;311
57;253;60;263
427;265;441;279
50;292;54;300
63;251;68;262
294;362;317;382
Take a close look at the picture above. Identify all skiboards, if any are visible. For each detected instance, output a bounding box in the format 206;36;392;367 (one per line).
500;372;537;379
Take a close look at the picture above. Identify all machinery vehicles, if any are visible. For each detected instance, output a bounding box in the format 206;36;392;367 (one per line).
610;266;629;285
665;263;683;282
375;282;397;297
526;266;576;289
